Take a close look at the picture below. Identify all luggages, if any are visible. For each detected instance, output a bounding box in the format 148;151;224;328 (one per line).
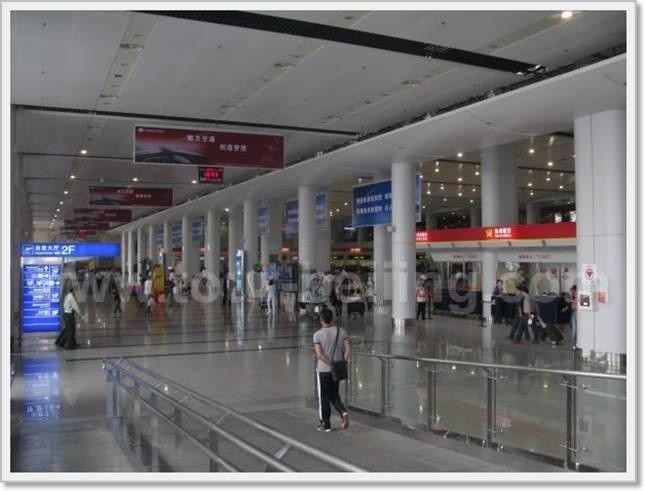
535;316;564;346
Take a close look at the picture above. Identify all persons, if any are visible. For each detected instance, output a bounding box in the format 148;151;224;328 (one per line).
313;310;349;432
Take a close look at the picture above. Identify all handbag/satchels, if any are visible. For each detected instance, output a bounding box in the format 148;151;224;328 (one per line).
330;360;348;382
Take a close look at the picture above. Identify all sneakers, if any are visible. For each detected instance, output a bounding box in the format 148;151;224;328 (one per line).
316;425;332;434
340;409;350;430
